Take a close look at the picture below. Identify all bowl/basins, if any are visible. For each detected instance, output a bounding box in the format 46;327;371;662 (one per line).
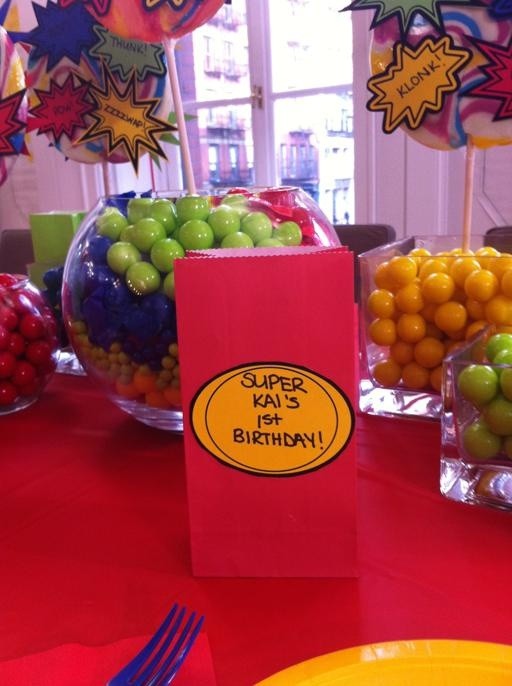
0;276;59;416
59;183;344;433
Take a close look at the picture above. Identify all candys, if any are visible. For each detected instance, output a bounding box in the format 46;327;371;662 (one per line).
0;25;28;188
27;40;169;199
84;0;227;196
367;1;512;254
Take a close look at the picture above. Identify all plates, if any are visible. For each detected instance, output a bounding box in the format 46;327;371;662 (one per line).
252;638;512;686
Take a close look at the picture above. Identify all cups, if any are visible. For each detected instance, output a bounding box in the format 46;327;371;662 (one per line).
357;232;512;426
438;324;512;513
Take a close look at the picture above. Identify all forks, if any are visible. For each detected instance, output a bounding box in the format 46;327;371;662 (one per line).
101;603;210;686
